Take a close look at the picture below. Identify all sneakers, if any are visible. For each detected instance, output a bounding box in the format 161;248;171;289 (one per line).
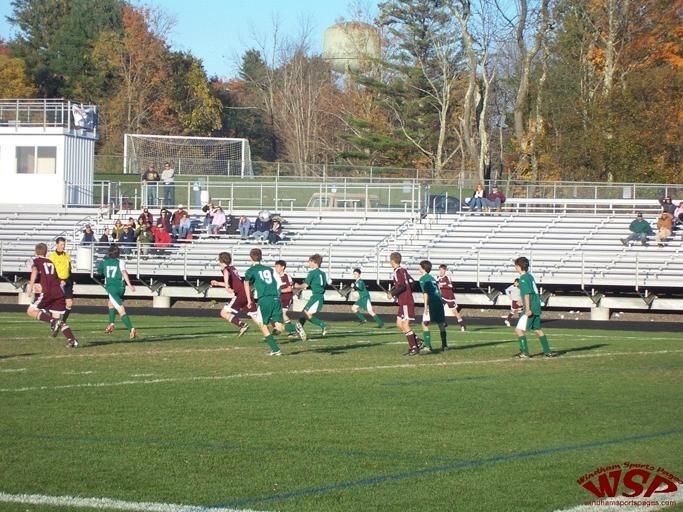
619;238;668;247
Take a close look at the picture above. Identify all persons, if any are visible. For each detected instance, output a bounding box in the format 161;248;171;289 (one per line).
512;256;555;360
418;261;448;355
207;249;334;357
26;244;79;349
346;268;383;328
465;184;486;212
81;203;283;254
385;253;424;357
619;195;683;248
97;244;137;339
503;279;525;328
481;186;506;213
160;162;175;208
47;237;74;328
438;264;466;332
141;164;160;209
